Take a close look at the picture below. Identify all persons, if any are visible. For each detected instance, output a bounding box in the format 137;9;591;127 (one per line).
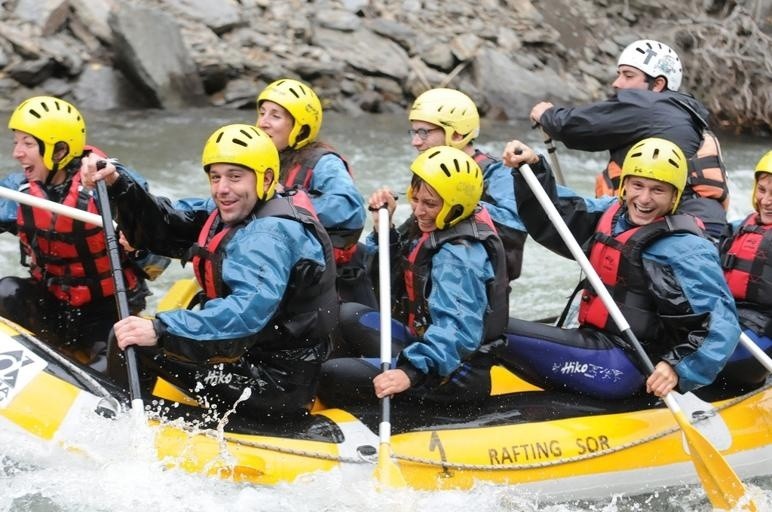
529;38;734;244
316;144;508;415
0;97;171;345
252;78;367;264
699;147;772;401
76;122;341;428
496;135;743;402
335;86;527;310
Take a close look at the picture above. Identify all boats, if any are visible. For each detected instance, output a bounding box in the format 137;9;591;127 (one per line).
0;305;762;504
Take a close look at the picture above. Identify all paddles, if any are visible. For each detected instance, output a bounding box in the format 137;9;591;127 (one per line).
515;154;756;512
369;195;412;488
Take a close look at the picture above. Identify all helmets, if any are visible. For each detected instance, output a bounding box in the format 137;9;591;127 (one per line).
752;151;772;211
619;138;688;214
618;40;683;91
257;79;323;151
409;88;479;150
407;146;484;231
8;96;86;172
202;124;281;201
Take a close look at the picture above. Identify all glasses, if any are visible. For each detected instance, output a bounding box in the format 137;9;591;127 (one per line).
409;127;439;141
